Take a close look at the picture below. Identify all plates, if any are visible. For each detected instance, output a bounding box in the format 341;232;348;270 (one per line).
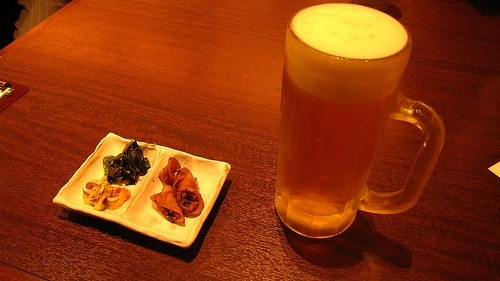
51;130;230;258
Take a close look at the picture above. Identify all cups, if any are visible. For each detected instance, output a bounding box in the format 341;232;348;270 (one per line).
275;3;446;239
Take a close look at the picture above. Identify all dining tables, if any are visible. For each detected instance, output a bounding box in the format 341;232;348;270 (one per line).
0;1;500;281
